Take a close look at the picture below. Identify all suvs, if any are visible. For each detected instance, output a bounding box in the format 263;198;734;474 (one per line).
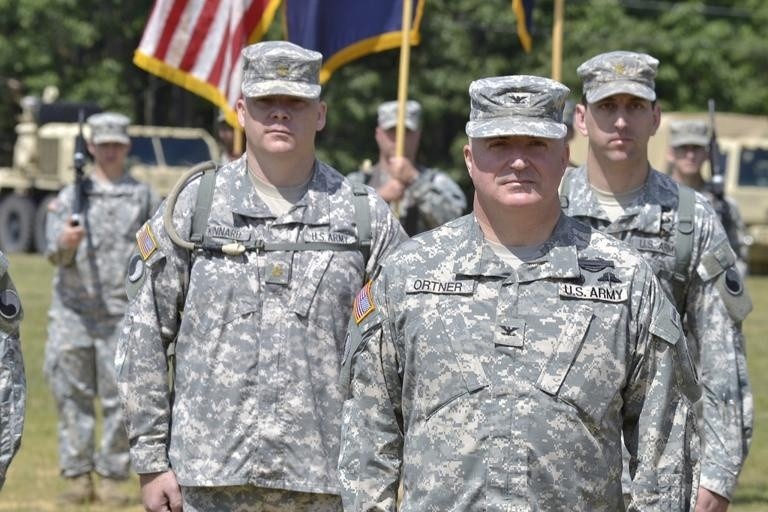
0;100;224;256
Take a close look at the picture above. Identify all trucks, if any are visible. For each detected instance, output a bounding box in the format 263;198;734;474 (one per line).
567;102;767;281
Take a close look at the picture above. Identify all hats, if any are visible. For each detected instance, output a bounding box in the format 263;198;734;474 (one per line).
240;38;325;102
464;73;572;143
668;118;714;147
85;110;134;147
375;99;425;133
575;49;662;106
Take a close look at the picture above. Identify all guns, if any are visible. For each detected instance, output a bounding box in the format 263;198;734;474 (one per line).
71;106;85;229
708;98;728;230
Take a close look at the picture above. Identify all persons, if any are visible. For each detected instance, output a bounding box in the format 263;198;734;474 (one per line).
665;118;753;278
116;40;416;511
338;74;706;512
344;99;470;239
556;50;756;511
1;251;27;484
44;112;167;506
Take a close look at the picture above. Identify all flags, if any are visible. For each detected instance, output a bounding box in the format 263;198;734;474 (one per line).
132;0;425;130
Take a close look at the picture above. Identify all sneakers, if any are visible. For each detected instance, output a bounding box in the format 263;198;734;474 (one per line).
53;476;130;509
